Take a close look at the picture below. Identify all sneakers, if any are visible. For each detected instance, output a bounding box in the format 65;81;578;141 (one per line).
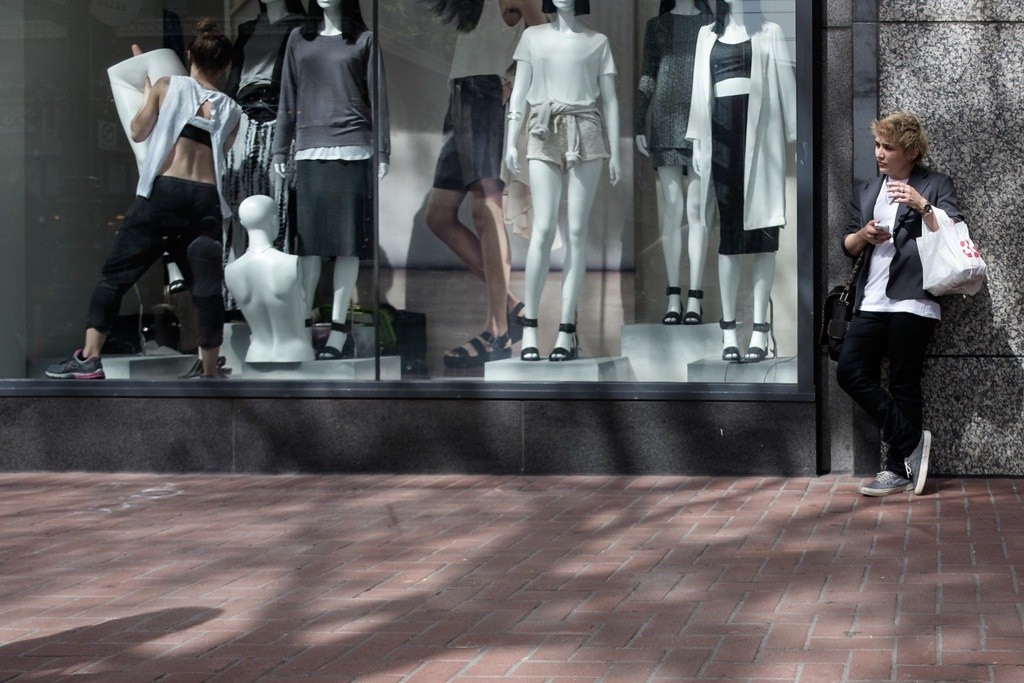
902;430;932;497
45;348;108;383
198;369;231;387
860;467;915;498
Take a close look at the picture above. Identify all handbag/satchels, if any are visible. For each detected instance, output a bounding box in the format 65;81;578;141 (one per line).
361;300;427;377
916;203;987;300
814;248;866;366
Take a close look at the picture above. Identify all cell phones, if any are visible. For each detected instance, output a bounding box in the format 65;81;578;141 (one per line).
874;222;890;235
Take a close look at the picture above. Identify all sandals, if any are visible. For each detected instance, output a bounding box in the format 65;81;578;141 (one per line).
717;317;741;362
743;321;772;364
548;323;576;362
515;315;543;363
683;289;706;325
316;320;354;358
661;284;682;326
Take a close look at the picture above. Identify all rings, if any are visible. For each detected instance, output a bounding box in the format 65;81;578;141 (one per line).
900;186;904;193
873;234;878;240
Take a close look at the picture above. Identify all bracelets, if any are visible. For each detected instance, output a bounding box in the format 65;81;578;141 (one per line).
507;110;524;123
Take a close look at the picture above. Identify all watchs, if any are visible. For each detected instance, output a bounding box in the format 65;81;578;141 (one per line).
919;200;933;218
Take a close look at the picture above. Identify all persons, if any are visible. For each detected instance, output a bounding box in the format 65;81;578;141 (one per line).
684;0;801;364
225;0;303;317
839;112;969;499
421;0;551;370
45;14;245;383
506;0;621;359
223;194;317;363
107;47;211;296
272;1;394;360
633;0;719;323
